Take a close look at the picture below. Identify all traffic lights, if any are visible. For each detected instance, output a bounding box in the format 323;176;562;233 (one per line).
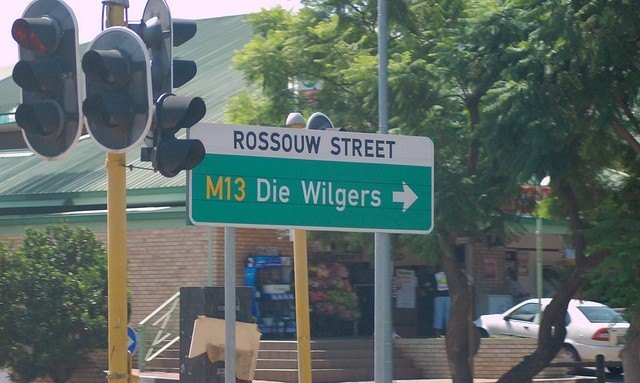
81;26;153;152
137;0;206;178
11;0;83;159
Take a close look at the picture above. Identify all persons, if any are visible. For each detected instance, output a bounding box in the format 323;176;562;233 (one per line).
430;265;451;338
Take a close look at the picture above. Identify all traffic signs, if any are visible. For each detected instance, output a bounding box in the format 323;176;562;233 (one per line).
188;122;434;235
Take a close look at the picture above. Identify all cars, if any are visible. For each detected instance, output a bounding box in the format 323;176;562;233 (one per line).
473;297;631;373
